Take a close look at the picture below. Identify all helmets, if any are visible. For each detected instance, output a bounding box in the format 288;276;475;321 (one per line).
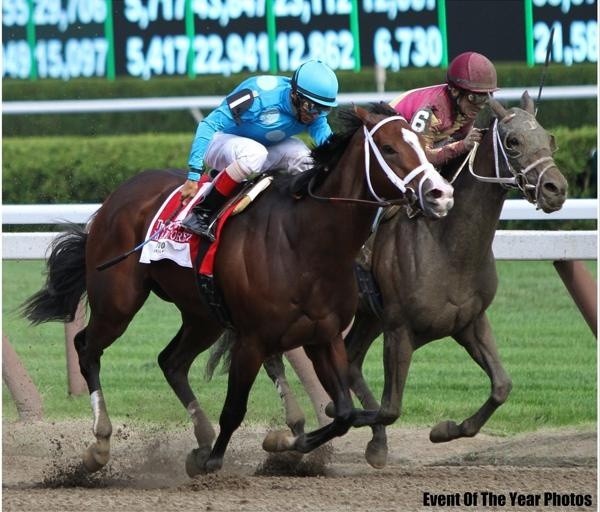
293;60;339;107
446;52;497;93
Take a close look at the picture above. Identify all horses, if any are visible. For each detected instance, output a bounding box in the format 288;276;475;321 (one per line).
204;90;569;470
4;100;455;474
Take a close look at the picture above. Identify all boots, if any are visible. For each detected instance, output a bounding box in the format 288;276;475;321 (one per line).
182;168;241;242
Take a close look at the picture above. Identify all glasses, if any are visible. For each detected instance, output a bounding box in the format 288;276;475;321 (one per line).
297;94;332;116
459;89;492;105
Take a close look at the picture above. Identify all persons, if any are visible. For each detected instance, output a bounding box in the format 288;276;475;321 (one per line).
177;59;340;243
388;51;500;168
